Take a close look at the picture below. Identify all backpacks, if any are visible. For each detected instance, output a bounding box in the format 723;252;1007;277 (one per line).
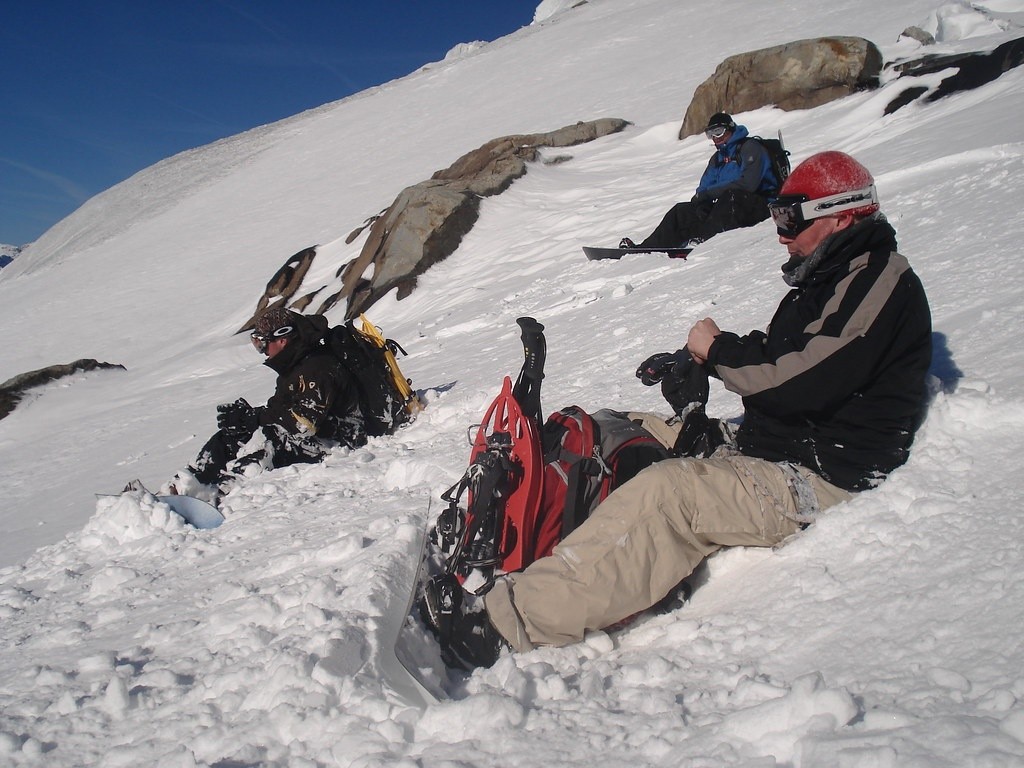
735;136;790;183
313;312;425;436
533;406;695;633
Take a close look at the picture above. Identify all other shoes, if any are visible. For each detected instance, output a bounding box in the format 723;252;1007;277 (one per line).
620;238;635;247
424;580;493;672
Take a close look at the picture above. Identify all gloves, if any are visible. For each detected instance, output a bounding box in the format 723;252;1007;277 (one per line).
217;397;265;446
691;191;710;210
635;343;710;417
697;200;713;222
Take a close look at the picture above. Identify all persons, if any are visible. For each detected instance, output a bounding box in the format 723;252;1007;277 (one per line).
168;308;369;500
414;150;933;671
619;110;782;249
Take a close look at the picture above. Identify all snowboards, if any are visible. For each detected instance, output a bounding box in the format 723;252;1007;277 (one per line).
97;484;225;528
582;246;694;262
395;493;446;708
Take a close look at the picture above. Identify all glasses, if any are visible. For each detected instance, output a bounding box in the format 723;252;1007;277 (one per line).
705;126;731;139
249;333;273;354
767;203;805;234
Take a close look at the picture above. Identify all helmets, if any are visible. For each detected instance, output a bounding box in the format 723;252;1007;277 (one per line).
256;309;305;341
782;151;880;217
704;113;737;132
